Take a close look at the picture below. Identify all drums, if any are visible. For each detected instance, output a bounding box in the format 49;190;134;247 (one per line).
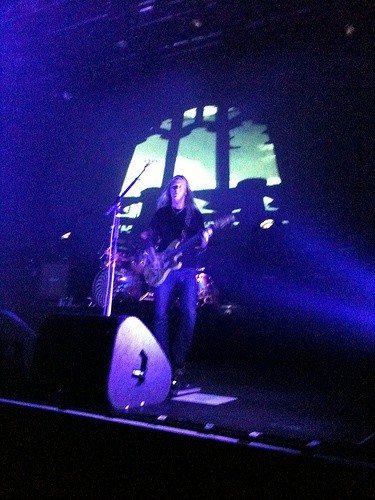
195;273;214;299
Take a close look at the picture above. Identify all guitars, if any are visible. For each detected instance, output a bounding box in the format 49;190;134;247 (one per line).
143;213;235;288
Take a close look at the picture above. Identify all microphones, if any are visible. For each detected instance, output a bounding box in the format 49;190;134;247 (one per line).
142;159;154;170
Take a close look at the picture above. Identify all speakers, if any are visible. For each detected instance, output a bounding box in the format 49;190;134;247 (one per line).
0;310;37;398
25;313;172;413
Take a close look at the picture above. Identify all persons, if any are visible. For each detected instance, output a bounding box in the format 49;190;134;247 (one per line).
131;175;213;372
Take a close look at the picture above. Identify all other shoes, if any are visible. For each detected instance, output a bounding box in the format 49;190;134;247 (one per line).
173;363;186;381
